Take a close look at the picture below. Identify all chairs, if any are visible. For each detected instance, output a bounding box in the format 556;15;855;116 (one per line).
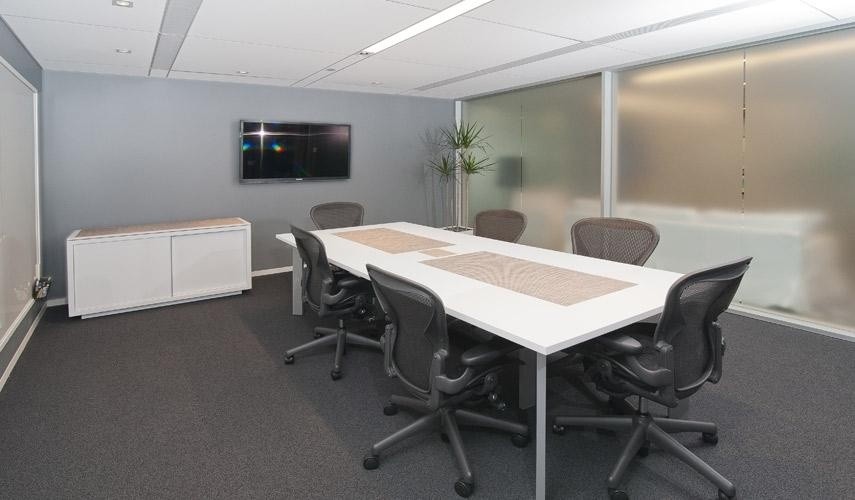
470;209;526;244
362;261;531;491
310;199;362;227
553;256;758;500
285;223;391;380
570;214;659;268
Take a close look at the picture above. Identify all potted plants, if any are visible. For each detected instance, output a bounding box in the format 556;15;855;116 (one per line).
424;120;496;235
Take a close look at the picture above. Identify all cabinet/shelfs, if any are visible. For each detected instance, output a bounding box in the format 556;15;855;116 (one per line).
65;216;255;323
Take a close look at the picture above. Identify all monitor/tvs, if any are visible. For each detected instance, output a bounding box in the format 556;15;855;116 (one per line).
239;120;351;185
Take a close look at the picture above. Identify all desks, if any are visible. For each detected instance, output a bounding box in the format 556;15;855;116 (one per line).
277;221;717;500
